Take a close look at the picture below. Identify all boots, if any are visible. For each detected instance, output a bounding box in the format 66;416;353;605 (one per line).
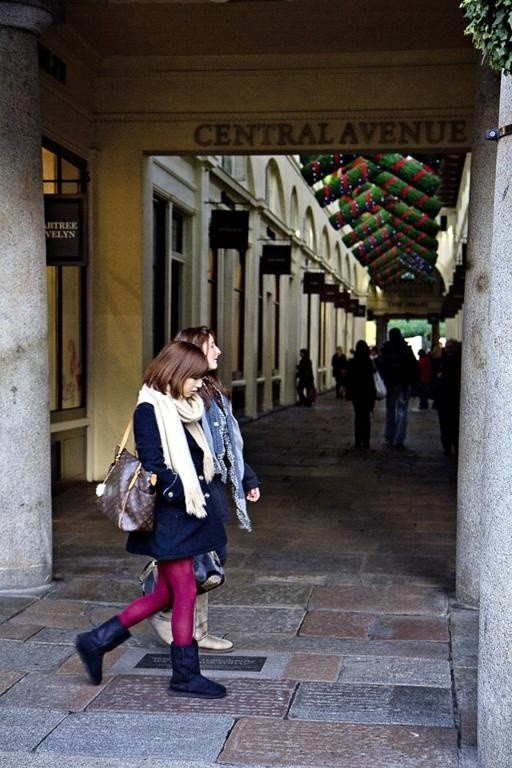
166;637;228;700
143;585;236;654
71;614;132;687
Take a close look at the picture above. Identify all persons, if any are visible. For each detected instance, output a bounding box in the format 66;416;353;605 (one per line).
71;339;230;700
377;327;418;450
294;347;316;409
331;342;443;409
430;338;462;461
346;340;375;451
139;322;265;654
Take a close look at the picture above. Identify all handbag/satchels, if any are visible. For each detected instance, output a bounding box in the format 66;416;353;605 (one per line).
92;442;160;534
139;547;226;598
371;369;388;401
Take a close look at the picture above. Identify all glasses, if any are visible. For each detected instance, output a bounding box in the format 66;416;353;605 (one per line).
197;326;211;339
